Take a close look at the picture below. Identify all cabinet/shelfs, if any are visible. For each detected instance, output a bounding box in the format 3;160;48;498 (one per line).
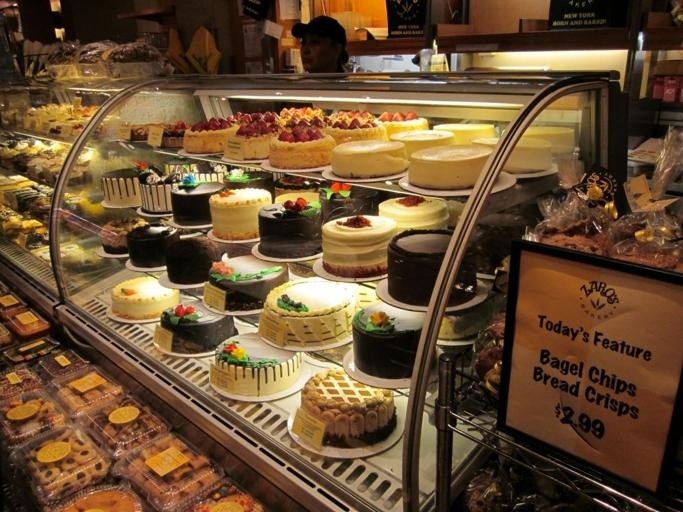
50;74;615;510
1;74;162;313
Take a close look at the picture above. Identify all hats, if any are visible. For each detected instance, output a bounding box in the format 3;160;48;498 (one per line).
291;15;345;41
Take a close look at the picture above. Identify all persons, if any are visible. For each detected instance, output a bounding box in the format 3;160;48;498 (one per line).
290;15;348;72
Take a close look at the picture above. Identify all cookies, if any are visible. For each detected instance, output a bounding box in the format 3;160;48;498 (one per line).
1;286;267;512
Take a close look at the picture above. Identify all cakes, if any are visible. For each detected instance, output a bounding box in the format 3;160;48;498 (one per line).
0;96;577;460
